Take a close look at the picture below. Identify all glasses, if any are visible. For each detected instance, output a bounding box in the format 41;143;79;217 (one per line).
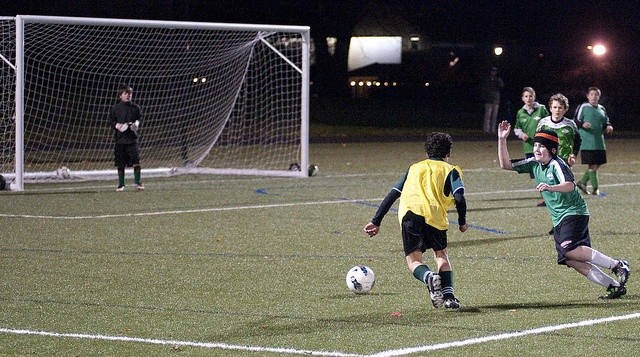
492;69;497;72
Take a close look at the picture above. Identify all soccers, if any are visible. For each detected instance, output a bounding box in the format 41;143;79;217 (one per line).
346;265;375;294
56;166;71;180
308;165;319;177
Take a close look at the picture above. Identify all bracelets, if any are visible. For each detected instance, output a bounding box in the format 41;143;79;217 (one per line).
115;123;120;129
135;119;140;127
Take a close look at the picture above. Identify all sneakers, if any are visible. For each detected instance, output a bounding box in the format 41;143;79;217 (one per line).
612;259;630;284
598;283;627;299
116;185;124;192
428;271;444;307
577;181;590;195
133;183;144;190
444;296;460;308
592;190;607;197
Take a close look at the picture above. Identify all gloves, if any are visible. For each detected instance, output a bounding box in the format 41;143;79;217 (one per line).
129;120;140;132
115;122;129;132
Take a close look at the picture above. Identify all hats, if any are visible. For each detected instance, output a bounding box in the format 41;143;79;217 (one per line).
533;130;559;157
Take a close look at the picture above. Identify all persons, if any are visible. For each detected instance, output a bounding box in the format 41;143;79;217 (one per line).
497;120;630;300
481;66;504;138
535;93;582;207
514;87;549;178
575;87;613;196
364;132;468;308
108;86;144;192
